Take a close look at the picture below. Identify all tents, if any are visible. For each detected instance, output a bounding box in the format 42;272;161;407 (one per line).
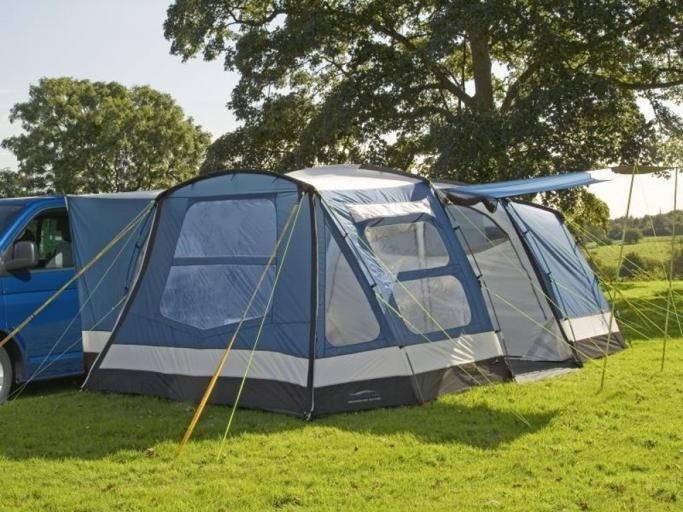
321;177;629;380
64;161;516;423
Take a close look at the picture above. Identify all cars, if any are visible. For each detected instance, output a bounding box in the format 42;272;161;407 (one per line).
0;195;85;403
